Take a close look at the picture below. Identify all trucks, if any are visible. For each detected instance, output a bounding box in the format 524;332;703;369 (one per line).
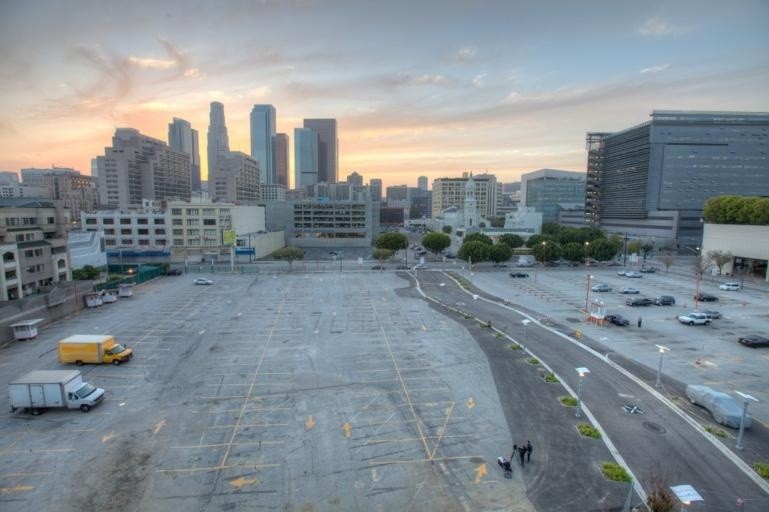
9;370;105;415
58;335;134;366
516;255;535;267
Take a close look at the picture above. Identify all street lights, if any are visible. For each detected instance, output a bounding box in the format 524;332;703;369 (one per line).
734;390;759;451
655;344;670;389
585;274;594;312
542;242;546;263
575;366;590;417
522;319;531;355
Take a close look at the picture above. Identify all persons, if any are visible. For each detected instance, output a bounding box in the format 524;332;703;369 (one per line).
637;315;642;328
526;439;533;461
518;444;527;465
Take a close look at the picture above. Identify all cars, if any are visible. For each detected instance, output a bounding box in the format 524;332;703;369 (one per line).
162;267;182;275
410;244;427;256
605;315;629;326
494;263;508;268
617;267;655;278
738;335;769;347
719;283;740;291
510;272;529;277
397;266;411;270
372;266;385;270
551;257;621;267
592;283;612;292
193;278;214;285
619;287;675;306
679;310;721;326
694;294;719;302
